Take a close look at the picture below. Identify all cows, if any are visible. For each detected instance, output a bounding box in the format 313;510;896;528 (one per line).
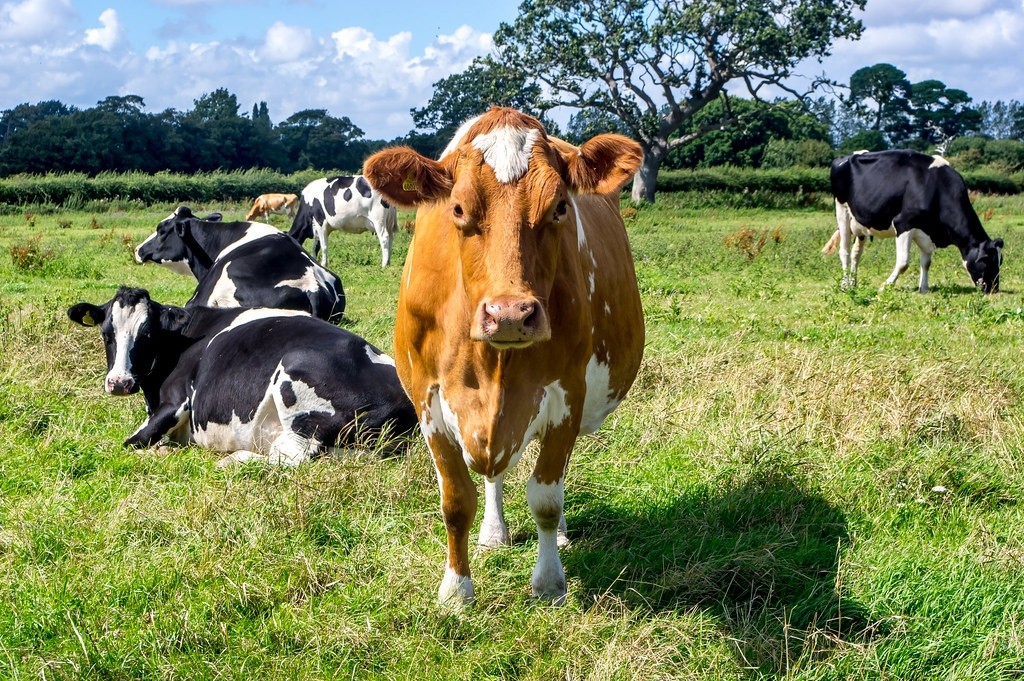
65;174;422;474
820;149;1005;294
360;105;649;613
